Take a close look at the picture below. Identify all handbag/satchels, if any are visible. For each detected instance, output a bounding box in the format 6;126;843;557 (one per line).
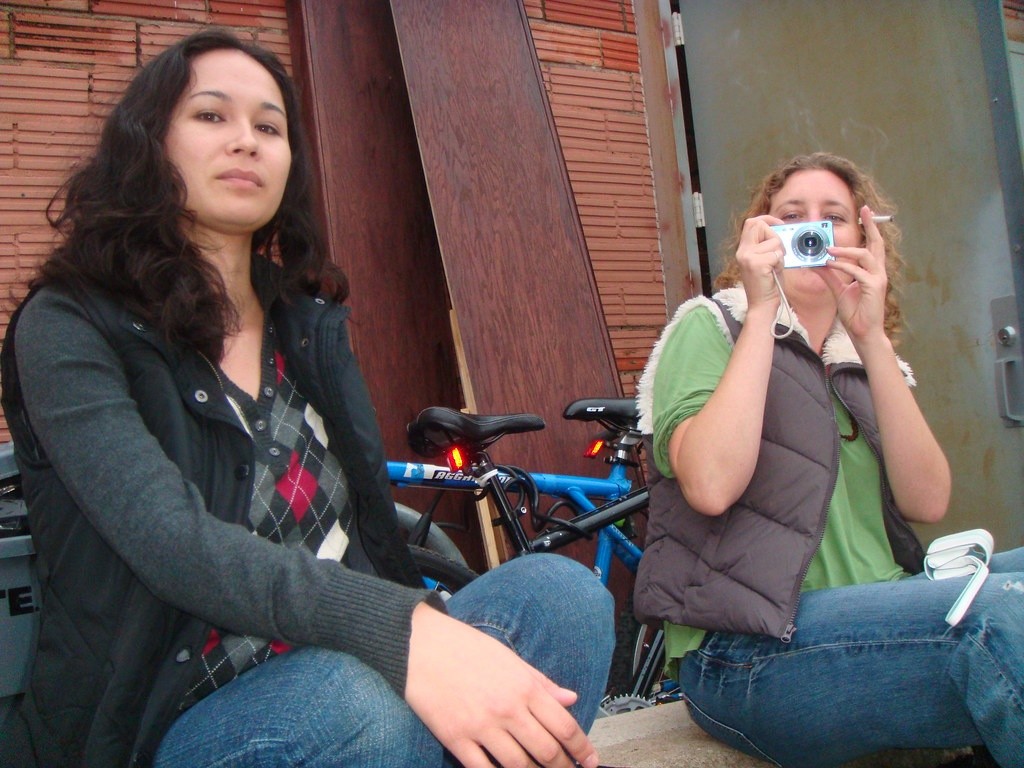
924;528;994;625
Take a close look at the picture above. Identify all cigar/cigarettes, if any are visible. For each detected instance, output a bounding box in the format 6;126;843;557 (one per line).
858;215;894;225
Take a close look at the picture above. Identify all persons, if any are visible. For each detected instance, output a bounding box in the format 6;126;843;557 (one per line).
631;151;1024;768
0;28;615;768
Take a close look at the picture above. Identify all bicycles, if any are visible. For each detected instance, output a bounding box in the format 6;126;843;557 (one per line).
385;398;688;722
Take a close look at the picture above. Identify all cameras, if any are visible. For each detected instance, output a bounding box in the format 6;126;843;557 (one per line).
770;220;835;269
